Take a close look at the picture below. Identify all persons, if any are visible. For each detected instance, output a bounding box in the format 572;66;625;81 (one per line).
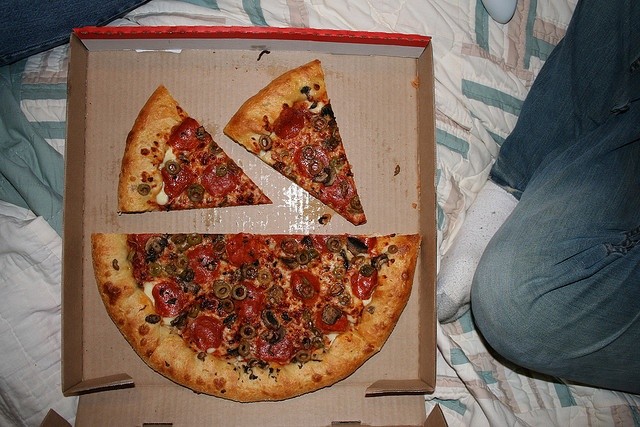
437;0;640;395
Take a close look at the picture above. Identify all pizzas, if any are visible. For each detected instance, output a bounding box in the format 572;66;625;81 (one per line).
222;59;367;225
90;233;423;404
116;83;273;217
